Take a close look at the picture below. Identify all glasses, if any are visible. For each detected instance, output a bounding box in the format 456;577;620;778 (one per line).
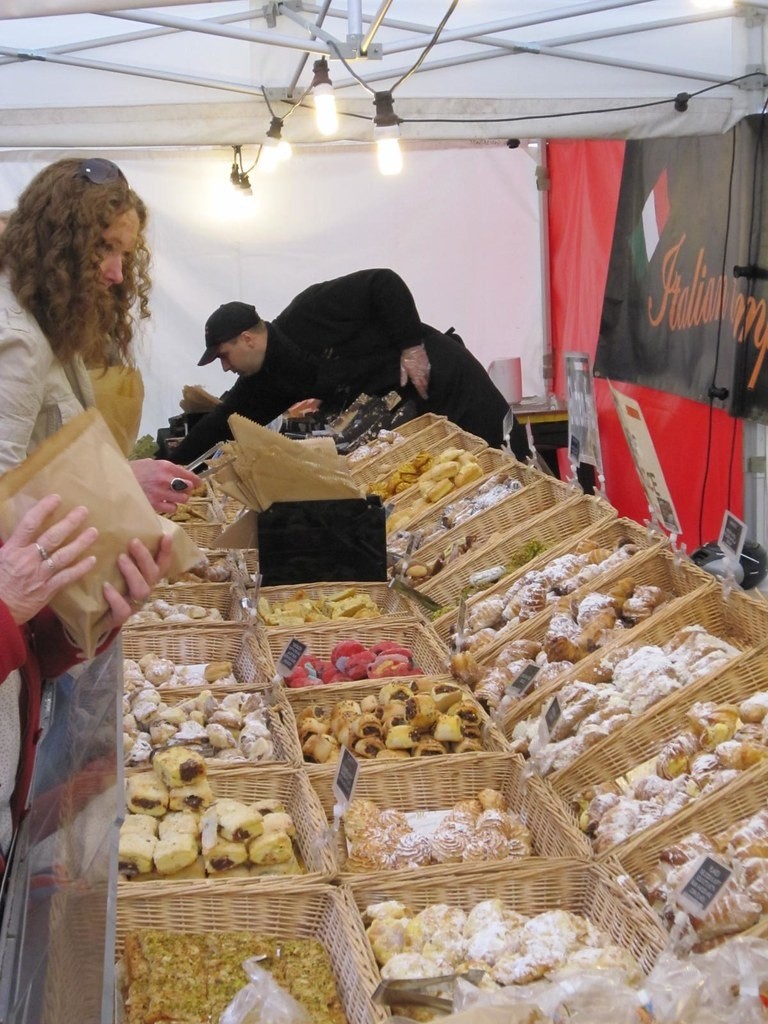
81;157;118;185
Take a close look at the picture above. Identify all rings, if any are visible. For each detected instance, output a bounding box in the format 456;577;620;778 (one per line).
33;542;50;559
169;478;190;492
131;596;148;608
47;558;56;572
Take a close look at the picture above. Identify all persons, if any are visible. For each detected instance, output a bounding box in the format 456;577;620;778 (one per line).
158;267;534;464
0;493;176;898
0;157;208;513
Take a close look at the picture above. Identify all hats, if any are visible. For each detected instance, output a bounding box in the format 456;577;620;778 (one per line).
197;302;260;366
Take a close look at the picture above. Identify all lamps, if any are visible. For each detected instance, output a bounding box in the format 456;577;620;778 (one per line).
311;59;339;135
224;162;256;210
259;117;284;166
372;91;403;177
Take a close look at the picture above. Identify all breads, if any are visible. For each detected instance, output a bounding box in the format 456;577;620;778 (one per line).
118;428;768;1024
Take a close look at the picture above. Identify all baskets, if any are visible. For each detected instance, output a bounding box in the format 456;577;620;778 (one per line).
51;412;768;1024
